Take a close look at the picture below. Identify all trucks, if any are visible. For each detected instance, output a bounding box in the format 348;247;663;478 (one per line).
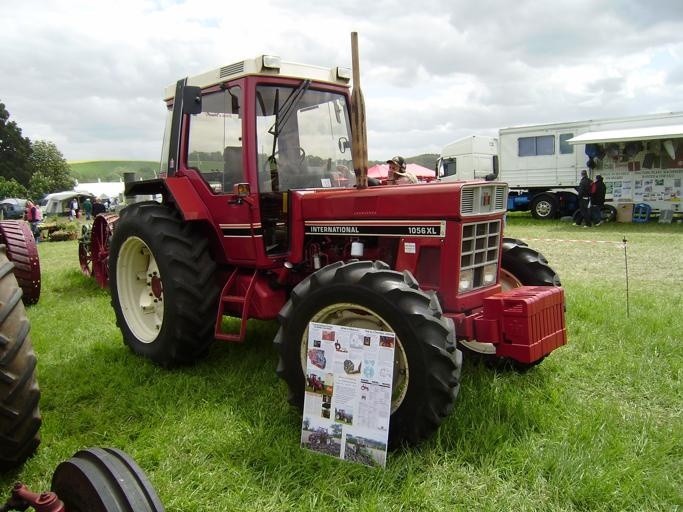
430;107;682;222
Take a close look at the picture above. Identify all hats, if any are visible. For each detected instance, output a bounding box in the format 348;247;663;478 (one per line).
386;155;407;168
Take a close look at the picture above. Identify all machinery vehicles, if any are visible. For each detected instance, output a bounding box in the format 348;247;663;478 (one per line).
106;50;569;458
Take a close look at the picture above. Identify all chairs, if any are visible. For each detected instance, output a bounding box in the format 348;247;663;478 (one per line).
222;145;245;192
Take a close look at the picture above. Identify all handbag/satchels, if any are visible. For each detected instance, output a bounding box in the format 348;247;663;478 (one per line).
591;182;597;194
31;225;42;238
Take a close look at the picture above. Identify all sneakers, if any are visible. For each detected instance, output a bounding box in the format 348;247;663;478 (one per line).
571;221;601;229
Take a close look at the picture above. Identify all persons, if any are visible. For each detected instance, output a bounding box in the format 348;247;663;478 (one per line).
337;157;407;187
591;174;606;227
392;170;418;185
572;168;591;228
5;192;124;243
